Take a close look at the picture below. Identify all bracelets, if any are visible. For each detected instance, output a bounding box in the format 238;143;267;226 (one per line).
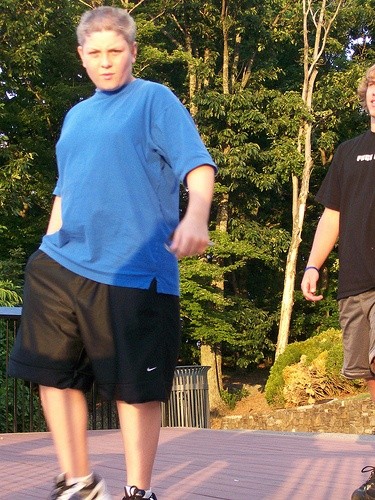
304;267;319;273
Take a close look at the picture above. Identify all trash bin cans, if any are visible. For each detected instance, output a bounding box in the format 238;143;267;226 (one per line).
166;364;212;429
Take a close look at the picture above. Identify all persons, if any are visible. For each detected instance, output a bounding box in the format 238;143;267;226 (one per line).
301;65;375;500
7;6;218;500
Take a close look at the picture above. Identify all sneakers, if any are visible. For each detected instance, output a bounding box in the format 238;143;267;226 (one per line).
121;484;156;500
48;473;105;500
351;466;375;500
